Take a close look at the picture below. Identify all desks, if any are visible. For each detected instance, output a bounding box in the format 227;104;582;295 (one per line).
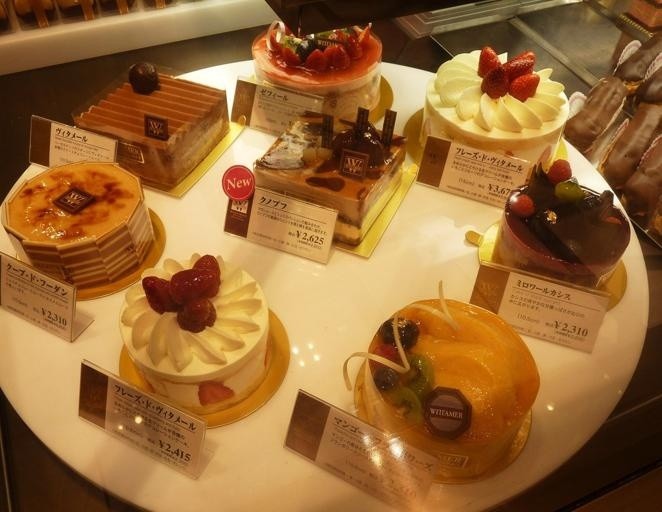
0;60;650;512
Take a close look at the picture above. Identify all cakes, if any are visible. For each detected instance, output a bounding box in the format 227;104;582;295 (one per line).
417;45;571;177
254;99;408;246
490;159;630;291
119;250;273;417
563;0;662;244
73;60;230;191
3;160;157;291
343;279;541;480
251;20;384;132
